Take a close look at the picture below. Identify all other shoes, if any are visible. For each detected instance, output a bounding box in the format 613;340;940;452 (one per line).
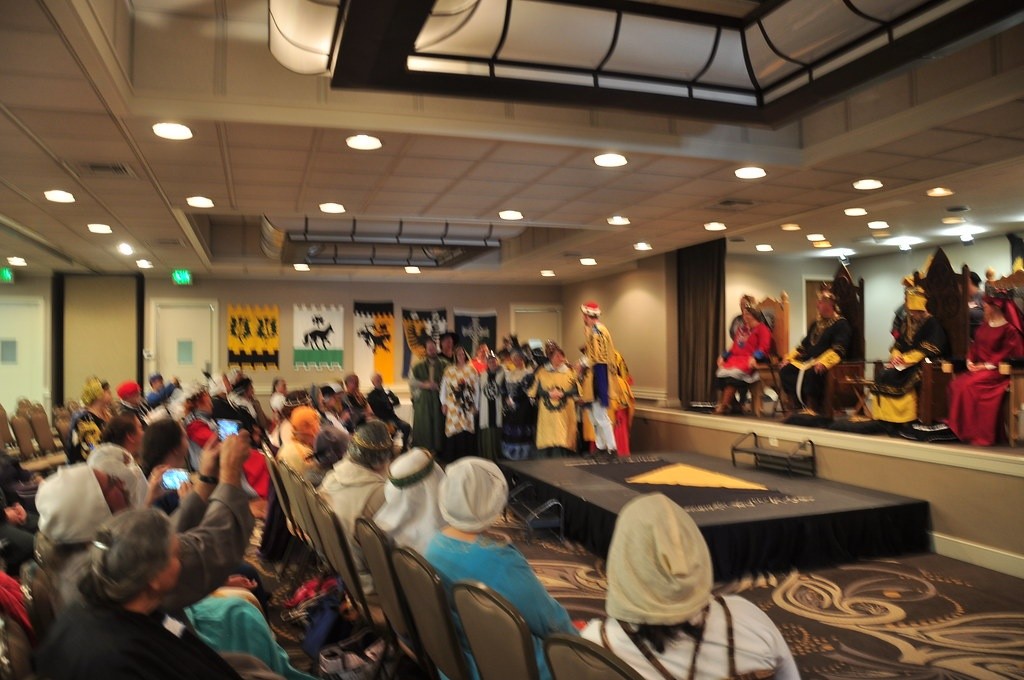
711;402;728;415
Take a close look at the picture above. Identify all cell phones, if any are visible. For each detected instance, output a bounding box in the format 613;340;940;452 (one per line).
216;418;243;440
160;467;191;489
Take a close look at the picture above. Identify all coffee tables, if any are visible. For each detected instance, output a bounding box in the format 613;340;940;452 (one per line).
835;375;875;423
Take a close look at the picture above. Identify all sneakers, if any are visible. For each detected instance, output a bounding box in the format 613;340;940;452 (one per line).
585;448;608;460
597;449;619;462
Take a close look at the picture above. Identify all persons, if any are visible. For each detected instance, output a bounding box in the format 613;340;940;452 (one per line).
776;290;854;427
956;272;985;340
0;302;804;679
941;288;1024;445
871;285;950;438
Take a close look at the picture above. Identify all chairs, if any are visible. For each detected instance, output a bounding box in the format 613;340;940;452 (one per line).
0;392;91;679
251;421;644;680
950;265;1024;450
778;260;867;423
871;245;969;437
726;292;798;417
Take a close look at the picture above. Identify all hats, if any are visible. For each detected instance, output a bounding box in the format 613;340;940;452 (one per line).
314;427;349;466
605;494;715;624
118;380;141;397
981;283;1016;307
150;373;163;382
289;407;318;445
35;466;115;543
904;285;927;311
437;455;509;532
330;383;343;392
581;302;601;317
815;281;835;303
